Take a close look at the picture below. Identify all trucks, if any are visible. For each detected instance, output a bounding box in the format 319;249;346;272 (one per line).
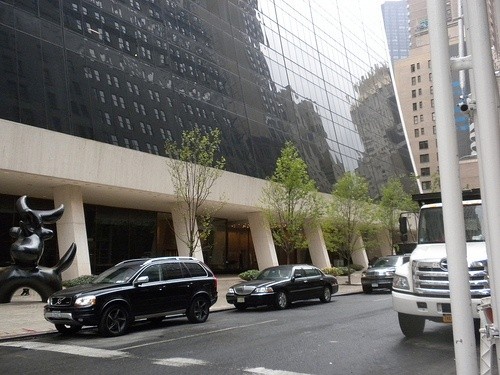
391;188;499;337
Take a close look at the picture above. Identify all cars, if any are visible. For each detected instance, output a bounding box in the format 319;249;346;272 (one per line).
225;263;340;311
360;255;406;294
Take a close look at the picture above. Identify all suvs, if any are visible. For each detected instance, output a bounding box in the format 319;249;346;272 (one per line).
43;256;219;337
